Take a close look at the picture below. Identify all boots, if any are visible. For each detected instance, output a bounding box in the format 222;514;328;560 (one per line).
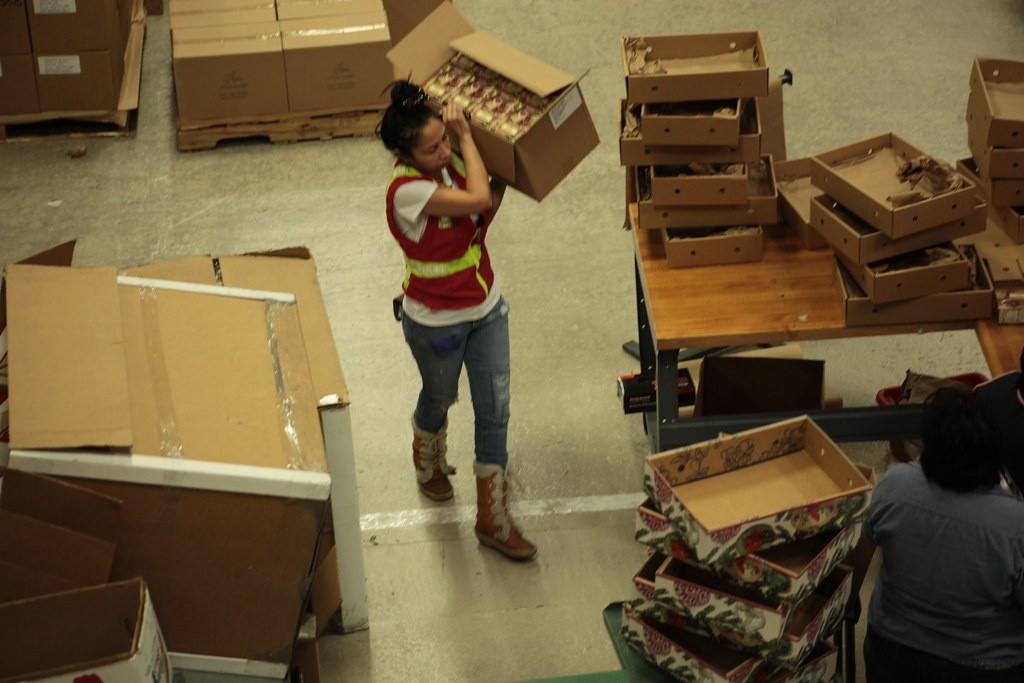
411;412;457;501
473;459;538;561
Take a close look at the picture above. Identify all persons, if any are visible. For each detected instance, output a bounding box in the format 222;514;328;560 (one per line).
846;382;1023;683
376;70;538;563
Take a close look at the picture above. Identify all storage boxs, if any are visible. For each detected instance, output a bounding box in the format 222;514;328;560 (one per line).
0;576;173;683
621;355;879;683
618;29;1024;327
0;0;602;203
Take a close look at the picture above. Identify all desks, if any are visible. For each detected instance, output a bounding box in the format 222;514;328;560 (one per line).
628;203;1024;453
